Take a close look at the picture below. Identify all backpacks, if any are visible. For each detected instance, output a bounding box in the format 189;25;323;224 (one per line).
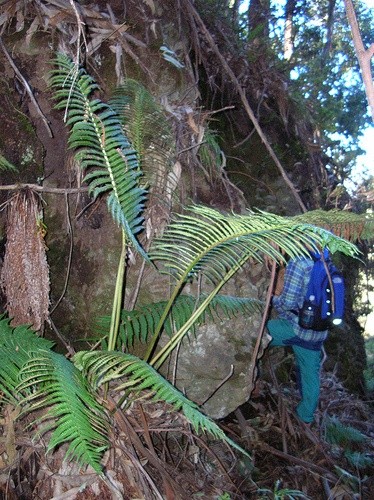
294;248;345;331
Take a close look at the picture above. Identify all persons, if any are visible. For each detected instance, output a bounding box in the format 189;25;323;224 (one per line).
266;228;339;424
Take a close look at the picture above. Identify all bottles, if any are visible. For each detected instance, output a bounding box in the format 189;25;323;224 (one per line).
299;295;317;329
